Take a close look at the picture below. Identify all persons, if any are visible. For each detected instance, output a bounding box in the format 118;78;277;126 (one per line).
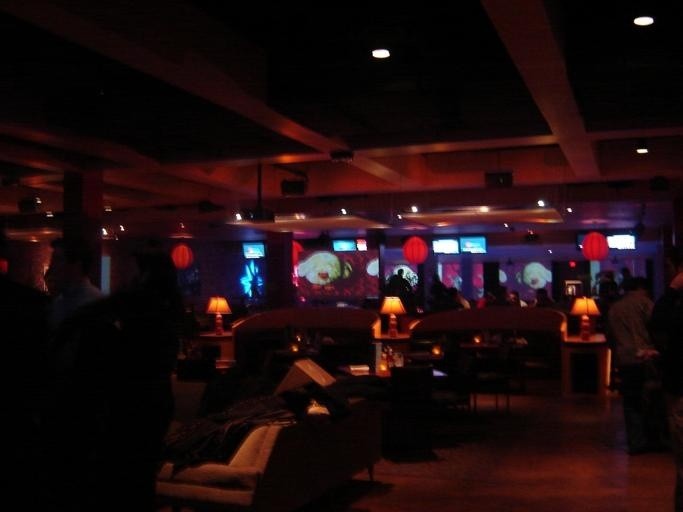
589;244;683;511
445;284;551;311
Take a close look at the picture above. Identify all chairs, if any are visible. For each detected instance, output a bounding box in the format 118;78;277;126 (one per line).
146;287;683;512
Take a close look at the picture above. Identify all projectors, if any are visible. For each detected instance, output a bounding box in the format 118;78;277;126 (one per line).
241;207;274;221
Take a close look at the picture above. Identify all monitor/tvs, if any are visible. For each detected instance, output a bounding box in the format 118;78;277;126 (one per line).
241;242;265;260
576;232;586;250
431;236;461;257
332;239;356;252
606;232;638;253
459;234;488;256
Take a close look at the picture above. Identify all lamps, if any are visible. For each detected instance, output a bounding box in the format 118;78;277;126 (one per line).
580;222;610;264
170;236;196;272
402;224;430;268
